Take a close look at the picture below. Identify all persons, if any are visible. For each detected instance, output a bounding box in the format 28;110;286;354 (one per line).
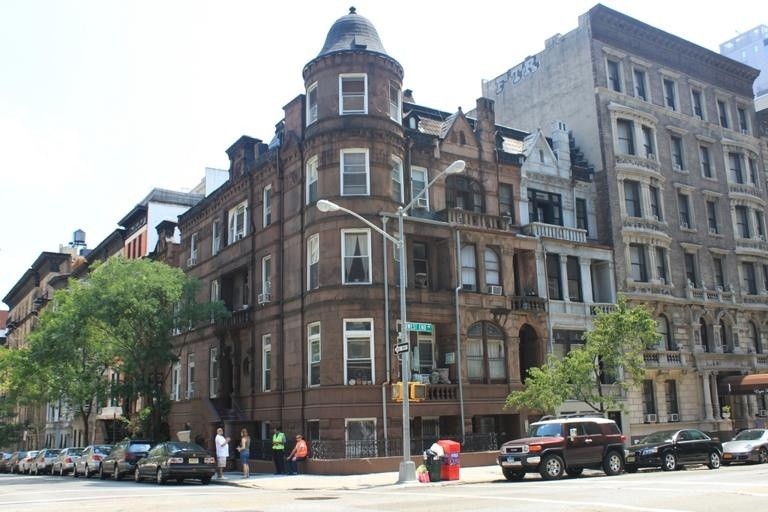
753;413;763;429
215;427;231;479
236;428;251;476
271;425;286;474
286;434;308;474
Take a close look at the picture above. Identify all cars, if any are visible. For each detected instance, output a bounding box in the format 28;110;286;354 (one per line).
622;425;725;472
0;445;115;479
130;439;218;486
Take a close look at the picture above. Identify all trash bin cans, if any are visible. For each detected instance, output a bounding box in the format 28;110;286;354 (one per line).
424;440;460;482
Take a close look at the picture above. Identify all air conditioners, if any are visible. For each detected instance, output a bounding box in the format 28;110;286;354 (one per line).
487;285;504;296
668;413;679;421
644;413;657;424
256;293;271;306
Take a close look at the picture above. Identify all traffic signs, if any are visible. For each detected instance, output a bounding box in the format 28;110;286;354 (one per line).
404;320;434;333
394;342;410;355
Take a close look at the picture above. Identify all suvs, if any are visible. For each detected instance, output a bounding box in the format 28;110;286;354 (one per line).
495;415;630;482
96;439;154;482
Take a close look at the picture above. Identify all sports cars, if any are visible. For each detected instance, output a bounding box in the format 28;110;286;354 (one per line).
719;427;768;467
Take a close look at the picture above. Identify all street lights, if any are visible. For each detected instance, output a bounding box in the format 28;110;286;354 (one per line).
313;159;473;487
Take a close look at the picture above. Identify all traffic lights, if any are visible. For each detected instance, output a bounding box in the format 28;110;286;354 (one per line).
391;385;400;401
413;384;426;400
